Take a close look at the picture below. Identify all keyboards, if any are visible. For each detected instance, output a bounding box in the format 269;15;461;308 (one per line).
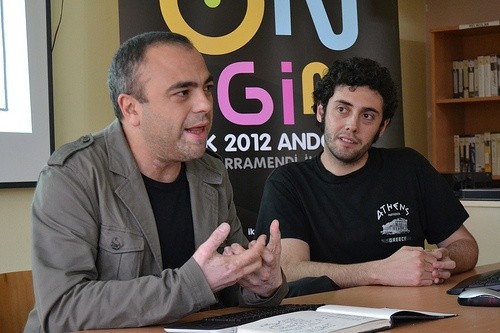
447;269;500;295
164;303;325;333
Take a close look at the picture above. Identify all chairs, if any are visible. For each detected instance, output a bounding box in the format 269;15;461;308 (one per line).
0;270;35;333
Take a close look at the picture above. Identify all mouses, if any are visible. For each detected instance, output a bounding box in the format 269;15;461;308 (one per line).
457;287;500;307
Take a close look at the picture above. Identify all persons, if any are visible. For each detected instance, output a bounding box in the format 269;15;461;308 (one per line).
251;54;479;287
24;33;290;333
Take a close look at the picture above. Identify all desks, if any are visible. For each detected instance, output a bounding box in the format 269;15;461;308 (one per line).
71;262;500;333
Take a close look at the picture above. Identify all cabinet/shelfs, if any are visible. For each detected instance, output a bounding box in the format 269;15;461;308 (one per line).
425;20;500;267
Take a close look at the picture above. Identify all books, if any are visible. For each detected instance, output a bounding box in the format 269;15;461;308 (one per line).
454;131;500;180
452;56;500;98
233;305;461;333
